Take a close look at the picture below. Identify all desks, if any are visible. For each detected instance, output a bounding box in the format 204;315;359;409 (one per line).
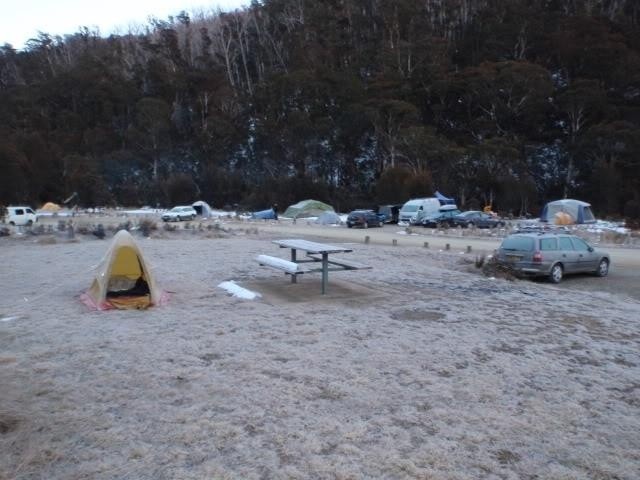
272;239;354;294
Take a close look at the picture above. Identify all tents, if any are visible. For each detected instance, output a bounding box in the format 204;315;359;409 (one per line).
77;228;172;317
284;198;335;222
540;200;597;225
313;209;342;225
192;200;211;218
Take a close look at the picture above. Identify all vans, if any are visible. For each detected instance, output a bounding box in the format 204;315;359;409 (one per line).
399;198;440;224
4;205;38;226
380;205;402;224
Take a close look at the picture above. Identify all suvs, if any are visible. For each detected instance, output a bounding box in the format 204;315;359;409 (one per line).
347;210;385;229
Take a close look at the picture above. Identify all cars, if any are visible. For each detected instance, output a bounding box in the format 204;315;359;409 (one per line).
446;210;505;228
498;233;611;283
421;210;460;227
161;205;196;222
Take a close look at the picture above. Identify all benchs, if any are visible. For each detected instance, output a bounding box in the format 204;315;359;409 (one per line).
307;254;372;271
259;257;312;275
